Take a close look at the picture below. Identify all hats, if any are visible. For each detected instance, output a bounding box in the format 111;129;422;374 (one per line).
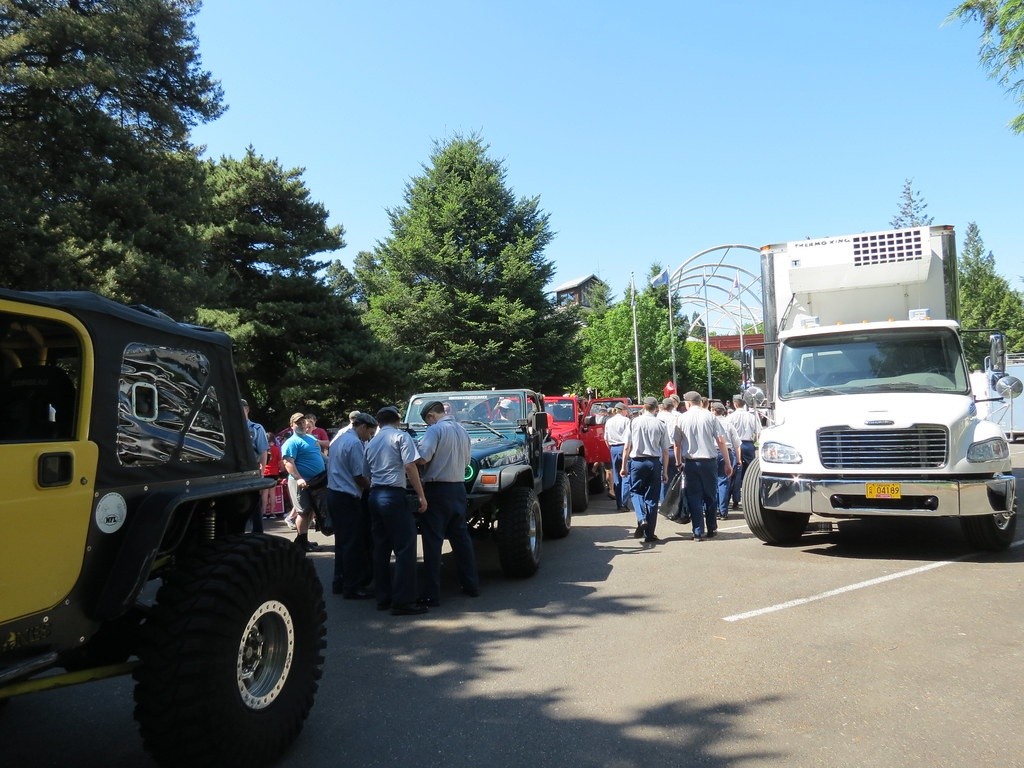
615;403;626;410
701;397;708;402
684;391;701;401
349;411;360;419
241;399;248;406
377;406;401;422
658;394;685;408
421;401;443;420
290;413;307;425
644;397;658;408
732;395;746;404
357;412;379;427
713;402;725;414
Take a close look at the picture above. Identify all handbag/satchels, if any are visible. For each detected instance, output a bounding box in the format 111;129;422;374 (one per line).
306;469;335;536
657;470;692;524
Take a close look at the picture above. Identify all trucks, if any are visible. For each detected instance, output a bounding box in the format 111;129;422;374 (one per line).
740;224;1022;555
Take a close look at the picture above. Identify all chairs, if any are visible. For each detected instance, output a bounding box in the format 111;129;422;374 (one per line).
4;365;75;439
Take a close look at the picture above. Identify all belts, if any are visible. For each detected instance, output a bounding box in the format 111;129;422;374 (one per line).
685;457;717;462
669;446;674;449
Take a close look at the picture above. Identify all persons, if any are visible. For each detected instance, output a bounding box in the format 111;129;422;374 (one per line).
497;399;514;420
590;390;756;542
241;399;480;615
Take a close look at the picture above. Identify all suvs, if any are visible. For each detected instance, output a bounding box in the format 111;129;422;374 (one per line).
629;405;645;418
497;396;632;512
395;389;574;578
0;289;327;768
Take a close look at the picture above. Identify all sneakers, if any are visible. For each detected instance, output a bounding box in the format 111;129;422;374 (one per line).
283;511;298;530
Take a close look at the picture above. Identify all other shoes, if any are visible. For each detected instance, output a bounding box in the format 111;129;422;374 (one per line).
634;520;648;539
294;534;320;552
353;589;378;600
392;607;430;615
269;513;277;519
590;468;599;477
376;602;391;610
732;503;739;510
416;595;440;607
263;514;267;519
608;492;616;500
716;512;728;519
309;517;317;529
692;534;704;542
617;504;630;512
707;529;717;538
463;586;481;597
644;535;659;542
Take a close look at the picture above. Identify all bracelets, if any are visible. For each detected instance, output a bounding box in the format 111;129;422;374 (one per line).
675;463;682;467
